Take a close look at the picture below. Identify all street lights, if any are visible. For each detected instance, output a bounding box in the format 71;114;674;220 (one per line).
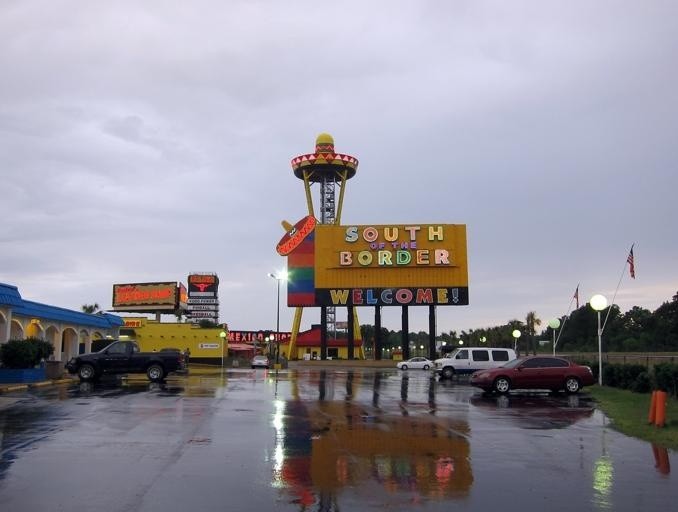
272;370;286;487
548;317;561;356
420;344;425;356
458;339;464;346
215;366;228;399
382;346;401;358
594;409;616;493
364;347;372;359
219;331;227;366
267;272;287;369
479;336;487;346
268;370;273;384
441;341;447;346
589;294;608;387
511;330;521;351
412;345;416;356
264;335;269;354
269;333;274;355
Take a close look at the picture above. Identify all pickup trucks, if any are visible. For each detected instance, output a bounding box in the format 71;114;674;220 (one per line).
67;383;182;403
64;339;187;382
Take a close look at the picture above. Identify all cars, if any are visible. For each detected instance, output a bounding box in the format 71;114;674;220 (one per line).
444;353;464;359
160;347;185;370
250;355;270;369
469;390;597;431
469;354;595;394
396;356;434;370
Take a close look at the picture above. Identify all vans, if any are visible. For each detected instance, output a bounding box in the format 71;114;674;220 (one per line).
433;347;516;380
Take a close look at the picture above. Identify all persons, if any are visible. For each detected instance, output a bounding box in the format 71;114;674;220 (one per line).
182;348;187;365
183;347;190;366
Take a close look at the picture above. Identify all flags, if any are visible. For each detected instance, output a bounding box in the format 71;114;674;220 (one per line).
626;246;636;280
573;284;579;310
